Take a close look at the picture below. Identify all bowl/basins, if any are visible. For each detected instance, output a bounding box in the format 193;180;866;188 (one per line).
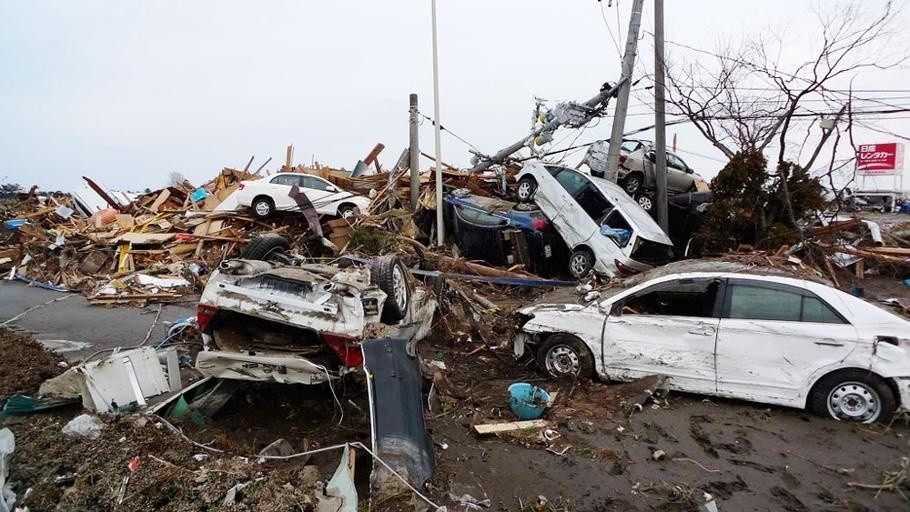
507;383;551;421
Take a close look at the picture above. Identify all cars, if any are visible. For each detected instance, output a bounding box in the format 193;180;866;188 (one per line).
237;171;373;220
192;225;447;388
512;258;910;425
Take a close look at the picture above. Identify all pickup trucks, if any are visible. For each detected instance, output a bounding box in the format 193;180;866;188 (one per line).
795;191;910;238
